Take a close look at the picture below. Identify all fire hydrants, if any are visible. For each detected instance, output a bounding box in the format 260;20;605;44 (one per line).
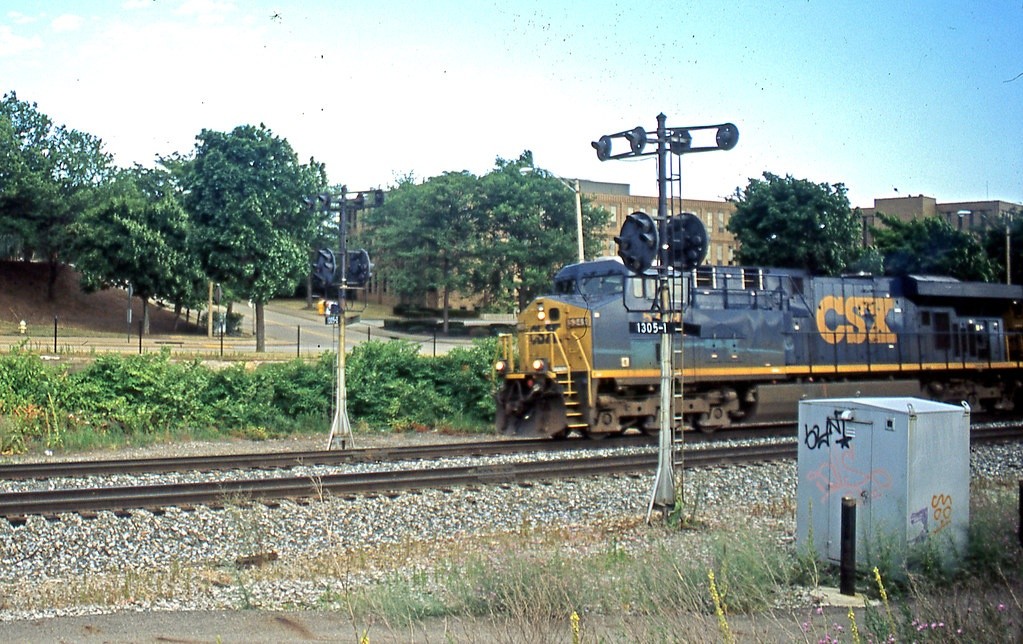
18;320;27;333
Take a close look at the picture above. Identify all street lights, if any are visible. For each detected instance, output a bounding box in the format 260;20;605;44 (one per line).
519;167;584;263
958;210;1012;287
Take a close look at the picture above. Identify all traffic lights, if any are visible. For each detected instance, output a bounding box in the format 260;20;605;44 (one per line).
312;248;336;283
665;213;711;271
348;249;374;286
613;211;658;274
303;193;332;211
592;127;647;162
345;189;385;210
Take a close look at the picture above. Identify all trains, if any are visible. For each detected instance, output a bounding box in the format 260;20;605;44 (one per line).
495;255;1023;438
